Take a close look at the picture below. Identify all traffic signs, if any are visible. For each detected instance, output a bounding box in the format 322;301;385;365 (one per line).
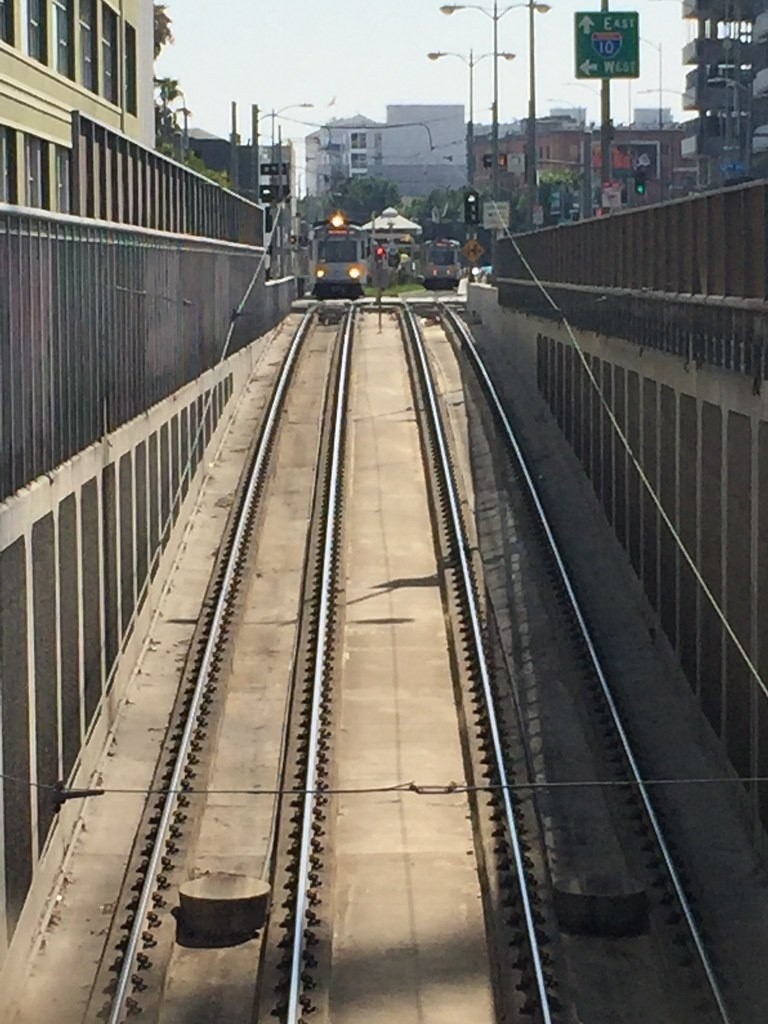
573;10;640;81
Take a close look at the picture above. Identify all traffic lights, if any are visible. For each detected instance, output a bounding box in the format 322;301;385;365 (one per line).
376;245;386;258
463;191;479;225
259;162;288;177
483;152;507;169
634;170;646;196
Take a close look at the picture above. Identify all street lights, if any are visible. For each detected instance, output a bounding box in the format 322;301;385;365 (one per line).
426;48;518;284
270;102;314;318
438;0;553;278
706;76;754;178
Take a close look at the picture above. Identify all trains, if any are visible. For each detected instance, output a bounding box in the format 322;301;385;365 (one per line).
306;208;369;301
418;236;461;289
